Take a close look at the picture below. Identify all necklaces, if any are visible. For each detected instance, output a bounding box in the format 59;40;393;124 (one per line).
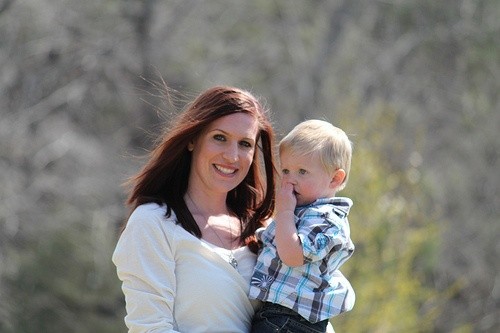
185;190;239;268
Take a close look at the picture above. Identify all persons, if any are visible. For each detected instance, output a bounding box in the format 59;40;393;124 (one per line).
249;119;355;333
112;87;335;333
